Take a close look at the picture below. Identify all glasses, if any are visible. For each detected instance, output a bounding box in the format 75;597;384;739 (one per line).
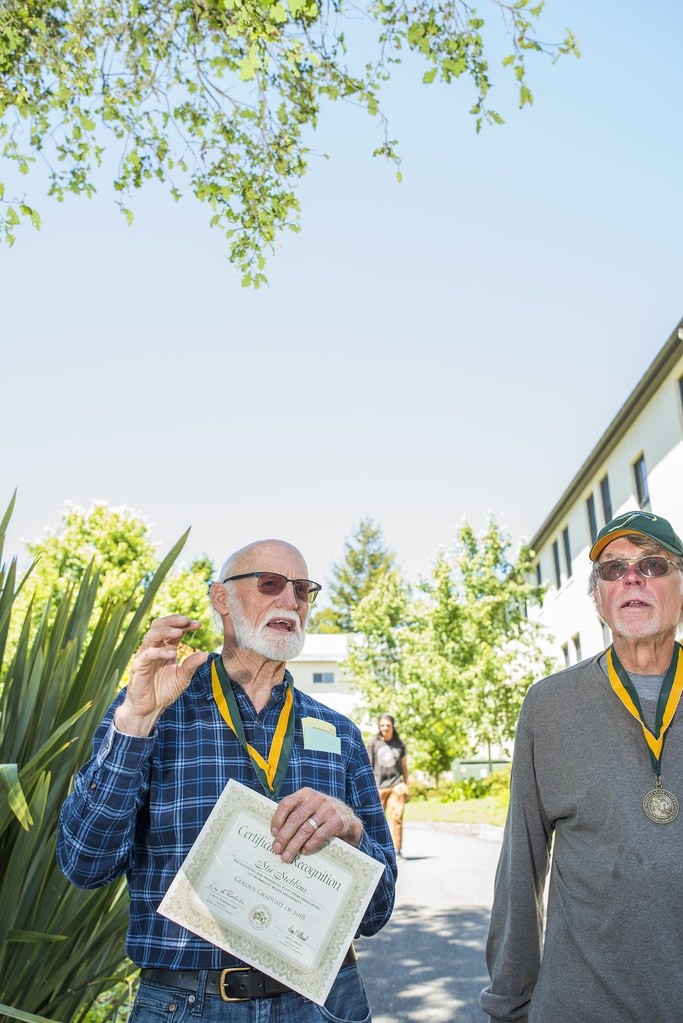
593;555;683;582
223;571;322;603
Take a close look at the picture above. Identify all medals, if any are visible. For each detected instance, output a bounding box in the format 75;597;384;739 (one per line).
642;789;680;824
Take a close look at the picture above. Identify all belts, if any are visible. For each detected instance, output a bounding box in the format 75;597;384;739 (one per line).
140;944;357;1002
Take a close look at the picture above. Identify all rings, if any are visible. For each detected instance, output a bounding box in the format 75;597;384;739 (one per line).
308;818;319;831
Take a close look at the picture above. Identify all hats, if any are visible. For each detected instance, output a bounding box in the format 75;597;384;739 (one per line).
589;511;683;563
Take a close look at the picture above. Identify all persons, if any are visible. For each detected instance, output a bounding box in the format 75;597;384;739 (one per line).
480;511;683;1023
57;540;398;1023
367;715;408;862
